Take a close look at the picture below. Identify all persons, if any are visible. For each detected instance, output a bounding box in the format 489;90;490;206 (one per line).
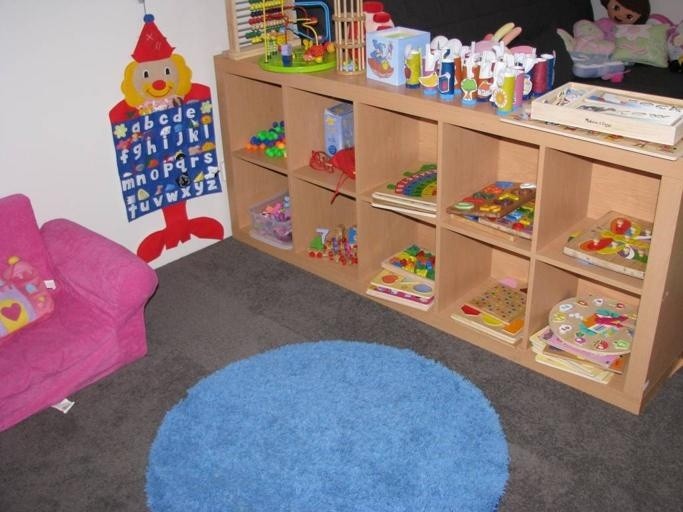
595;1;651;44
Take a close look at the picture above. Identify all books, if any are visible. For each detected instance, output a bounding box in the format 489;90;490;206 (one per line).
529;327;625;383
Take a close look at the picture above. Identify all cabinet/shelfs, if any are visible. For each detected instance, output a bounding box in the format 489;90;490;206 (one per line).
211;50;682;417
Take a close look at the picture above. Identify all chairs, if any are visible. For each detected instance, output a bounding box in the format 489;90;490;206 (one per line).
0;194;157;434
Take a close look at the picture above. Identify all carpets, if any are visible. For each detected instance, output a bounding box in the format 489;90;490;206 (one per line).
144;338;511;512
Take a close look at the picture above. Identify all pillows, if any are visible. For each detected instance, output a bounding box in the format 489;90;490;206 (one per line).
1;257;56;343
609;22;669;69
